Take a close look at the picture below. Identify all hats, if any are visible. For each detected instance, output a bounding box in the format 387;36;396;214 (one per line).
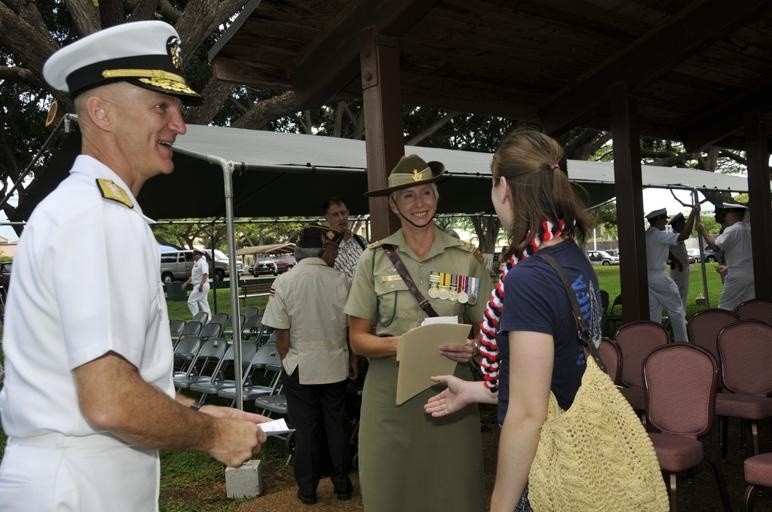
721;202;749;211
645;208;669;222
191;245;205;255
288;225;330;249
668;212;686;225
363;154;446;197
42;19;202;104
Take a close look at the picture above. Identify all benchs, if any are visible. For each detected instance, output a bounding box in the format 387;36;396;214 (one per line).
238;282;272;307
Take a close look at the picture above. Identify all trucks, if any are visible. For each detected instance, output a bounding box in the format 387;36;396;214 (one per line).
684;237;702;265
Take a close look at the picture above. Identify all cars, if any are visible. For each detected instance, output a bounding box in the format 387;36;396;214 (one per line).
249;263;254;276
253;256;278;276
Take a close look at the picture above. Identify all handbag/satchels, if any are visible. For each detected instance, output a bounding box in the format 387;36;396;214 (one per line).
528;251;670;511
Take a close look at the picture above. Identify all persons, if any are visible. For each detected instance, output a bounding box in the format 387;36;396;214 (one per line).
341;153;499;512
260;223;355;505
181;247;212;325
712;202;731;285
668;211;690;328
323;196;370;278
424;126;671;512
645;202;701;342
694;201;755;312
0;20;274;512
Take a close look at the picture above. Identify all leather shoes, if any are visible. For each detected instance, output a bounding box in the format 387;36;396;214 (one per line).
297;489;317;505
337;492;351;500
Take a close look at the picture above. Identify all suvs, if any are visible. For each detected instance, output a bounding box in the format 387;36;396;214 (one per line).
605;249;617;257
702;243;720;263
0;260;10;287
272;254;297;273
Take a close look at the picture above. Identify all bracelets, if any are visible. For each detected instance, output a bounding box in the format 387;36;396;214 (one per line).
189;401;203;411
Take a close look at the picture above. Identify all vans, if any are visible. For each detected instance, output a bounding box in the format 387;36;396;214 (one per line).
160;248;244;283
588;250;620;265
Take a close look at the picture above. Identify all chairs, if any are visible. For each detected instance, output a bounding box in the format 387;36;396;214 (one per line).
166;306;298;469
585;287;772;512
